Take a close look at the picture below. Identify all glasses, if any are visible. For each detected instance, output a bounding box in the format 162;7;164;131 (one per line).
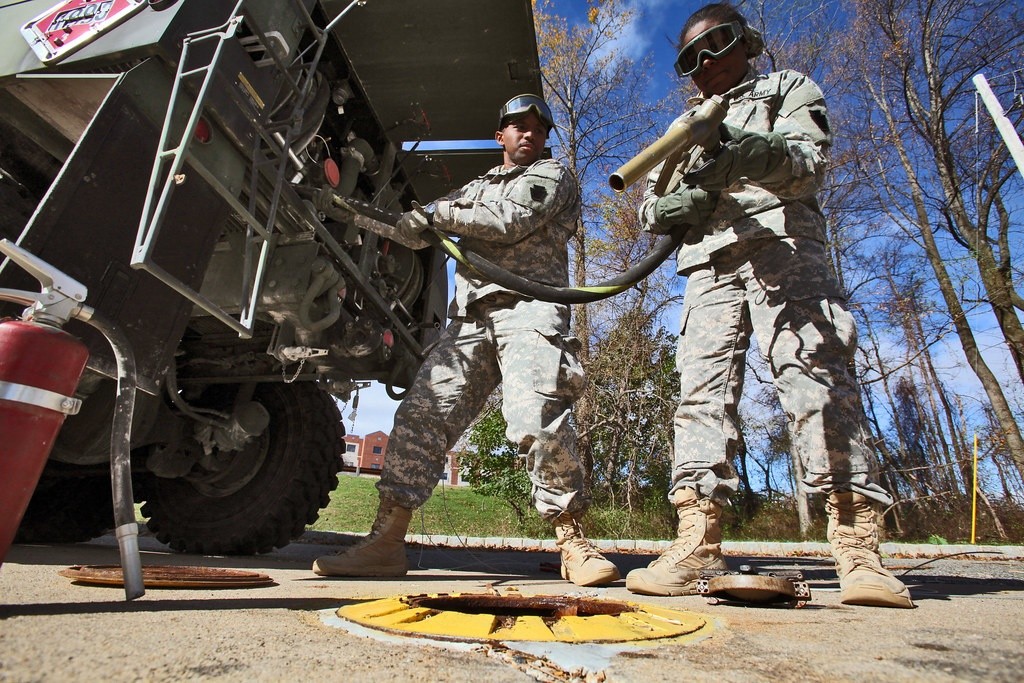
673;20;743;77
504;93;553;126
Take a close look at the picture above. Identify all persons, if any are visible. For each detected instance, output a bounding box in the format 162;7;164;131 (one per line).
311;92;621;587
626;5;913;607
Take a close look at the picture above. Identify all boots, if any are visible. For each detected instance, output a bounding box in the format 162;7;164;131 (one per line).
312;499;412;577
549;508;620;586
826;490;913;608
626;488;727;596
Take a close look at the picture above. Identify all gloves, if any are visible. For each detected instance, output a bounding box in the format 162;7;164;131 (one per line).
681;115;788;192
395;200;431;240
654;187;719;233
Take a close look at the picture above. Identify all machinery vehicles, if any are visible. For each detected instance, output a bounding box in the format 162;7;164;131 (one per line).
0;0;546;556
